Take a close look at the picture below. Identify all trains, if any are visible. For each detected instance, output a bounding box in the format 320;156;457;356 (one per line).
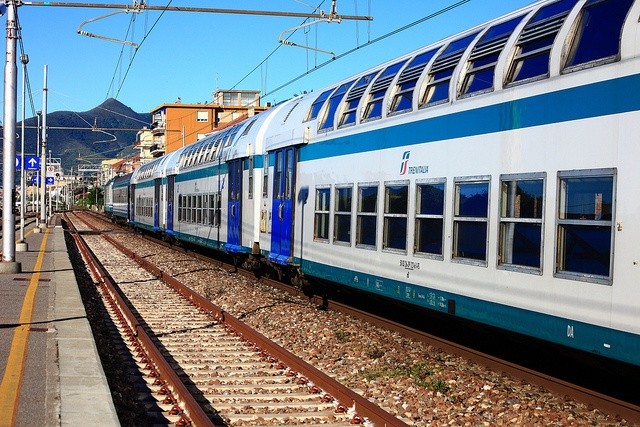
103;0;640;366
